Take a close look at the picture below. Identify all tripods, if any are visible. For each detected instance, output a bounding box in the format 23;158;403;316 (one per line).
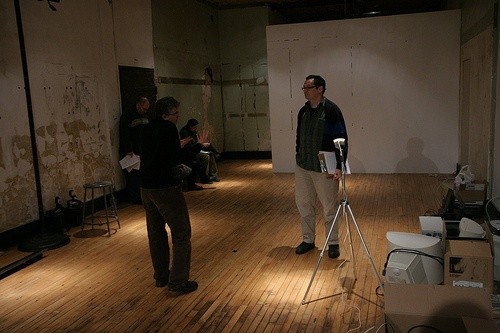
302;145;385;305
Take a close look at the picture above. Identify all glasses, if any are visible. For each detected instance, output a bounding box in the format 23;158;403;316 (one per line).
165;113;179;117
302;86;316;91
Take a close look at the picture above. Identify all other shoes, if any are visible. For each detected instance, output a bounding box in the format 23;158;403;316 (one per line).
212;176;219;182
296;241;315;255
328;244;339;258
169;280;198;297
156;270;171;287
187;184;202;190
200;179;211;183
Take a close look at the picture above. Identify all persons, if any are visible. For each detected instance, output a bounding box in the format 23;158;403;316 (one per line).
137;94;210;294
120;96;151;206
294;75;349;258
174;118;220;191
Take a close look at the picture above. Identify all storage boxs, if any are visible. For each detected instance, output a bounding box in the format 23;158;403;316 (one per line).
385;282;493;333
439;217;493;295
454;183;484;203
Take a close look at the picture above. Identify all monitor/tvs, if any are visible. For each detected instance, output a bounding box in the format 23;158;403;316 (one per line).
384;231;445;287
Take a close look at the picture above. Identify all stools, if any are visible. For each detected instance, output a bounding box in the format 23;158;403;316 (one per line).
81;181;121;237
